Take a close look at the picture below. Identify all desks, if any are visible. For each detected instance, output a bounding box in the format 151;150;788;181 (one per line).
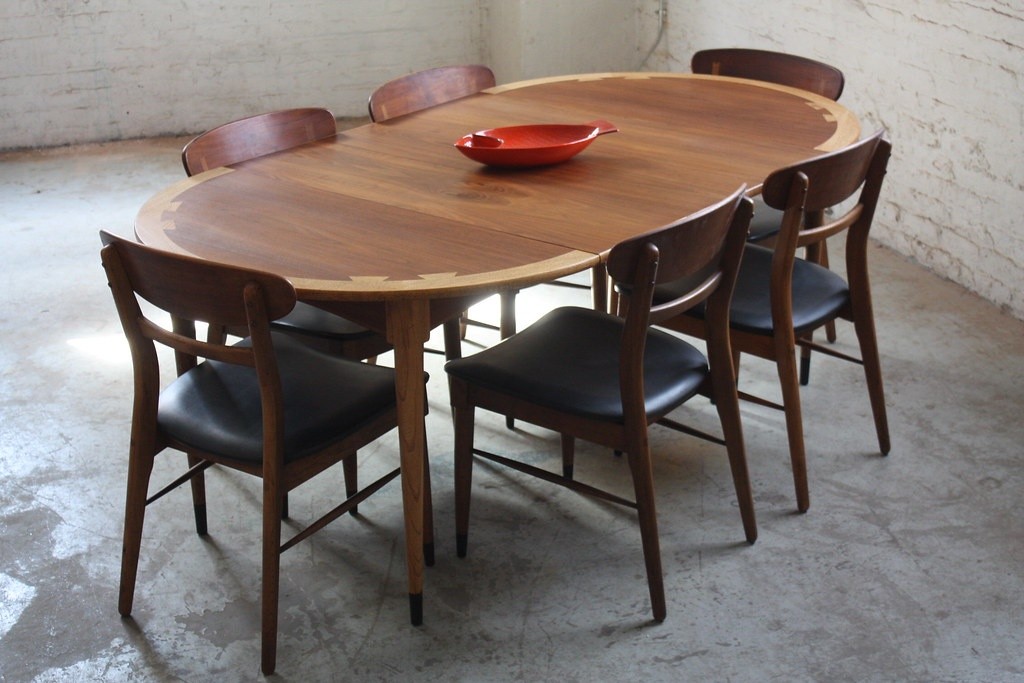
135;70;862;568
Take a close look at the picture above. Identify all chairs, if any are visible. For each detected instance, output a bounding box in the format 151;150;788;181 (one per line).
691;48;847;341
443;181;757;623
614;125;893;512
100;228;432;677
184;105;448;517
366;61;607;429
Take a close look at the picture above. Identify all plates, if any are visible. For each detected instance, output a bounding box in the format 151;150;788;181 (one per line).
452;118;620;167
